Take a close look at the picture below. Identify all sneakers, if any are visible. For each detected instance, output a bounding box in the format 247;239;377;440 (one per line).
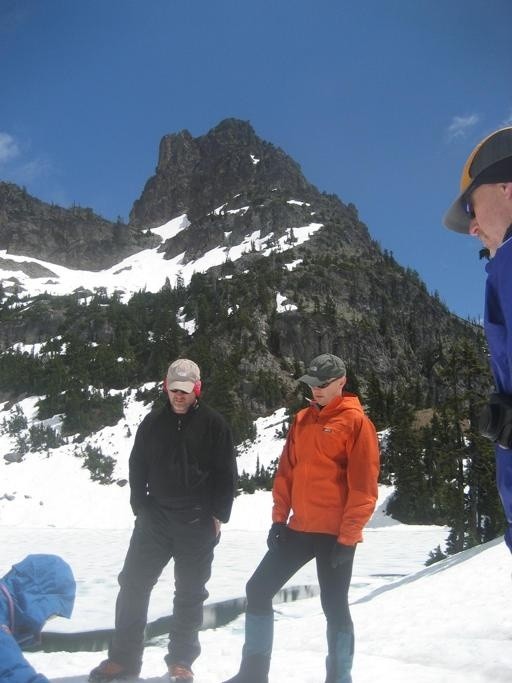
85;657;144;682
164;653;195;682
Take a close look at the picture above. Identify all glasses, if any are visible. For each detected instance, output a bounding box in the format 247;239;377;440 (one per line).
459;177;505;218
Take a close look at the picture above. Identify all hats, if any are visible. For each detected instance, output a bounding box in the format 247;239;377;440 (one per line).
164;359;201;393
298;351;347;387
441;124;512;233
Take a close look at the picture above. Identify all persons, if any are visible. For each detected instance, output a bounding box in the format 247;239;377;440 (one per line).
87;359;239;683
444;125;512;555
0;554;76;683
222;353;380;683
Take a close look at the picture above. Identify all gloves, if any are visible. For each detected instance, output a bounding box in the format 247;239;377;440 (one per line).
266;523;290;560
328;542;356;568
